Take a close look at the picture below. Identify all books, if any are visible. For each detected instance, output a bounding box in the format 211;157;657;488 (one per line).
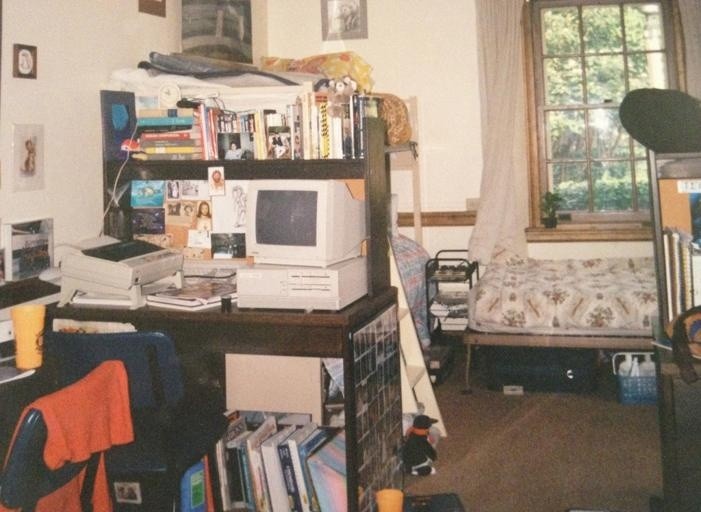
144;296;239;311
146;280;237;308
199;409;348;512
652;226;701;362
100;88;382;160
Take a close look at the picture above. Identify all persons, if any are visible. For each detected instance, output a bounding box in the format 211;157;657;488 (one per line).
165;170;247;259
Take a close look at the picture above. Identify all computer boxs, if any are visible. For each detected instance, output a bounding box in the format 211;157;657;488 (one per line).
235;255;368;312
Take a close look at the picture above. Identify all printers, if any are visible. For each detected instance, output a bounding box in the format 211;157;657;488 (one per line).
56;235;185;309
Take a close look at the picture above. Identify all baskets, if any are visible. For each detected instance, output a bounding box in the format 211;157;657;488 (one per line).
612;352;659;405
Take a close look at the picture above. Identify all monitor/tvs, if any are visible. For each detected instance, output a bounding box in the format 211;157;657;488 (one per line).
243;177;367;268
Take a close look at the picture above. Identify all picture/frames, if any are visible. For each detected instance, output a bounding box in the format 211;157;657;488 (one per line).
321;0;368;41
13;44;37;79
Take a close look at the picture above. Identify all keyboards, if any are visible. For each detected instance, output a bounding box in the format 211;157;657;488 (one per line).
182;258;235;278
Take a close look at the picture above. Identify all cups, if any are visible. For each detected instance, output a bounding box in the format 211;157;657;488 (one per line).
10;304;47;370
374;488;404;512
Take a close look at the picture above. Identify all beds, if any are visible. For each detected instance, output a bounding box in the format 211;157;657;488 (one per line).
462;249;659;394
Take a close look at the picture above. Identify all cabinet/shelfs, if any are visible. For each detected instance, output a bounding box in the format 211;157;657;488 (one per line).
101;90;391;298
647;148;701;512
426;250;478;346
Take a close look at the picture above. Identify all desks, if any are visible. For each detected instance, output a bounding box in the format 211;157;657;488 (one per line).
44;286;406;511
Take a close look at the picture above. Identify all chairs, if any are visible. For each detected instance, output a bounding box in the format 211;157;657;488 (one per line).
0;358;135;510
42;329;229;512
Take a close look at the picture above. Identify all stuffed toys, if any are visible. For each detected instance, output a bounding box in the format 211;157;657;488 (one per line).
400;413;443;477
